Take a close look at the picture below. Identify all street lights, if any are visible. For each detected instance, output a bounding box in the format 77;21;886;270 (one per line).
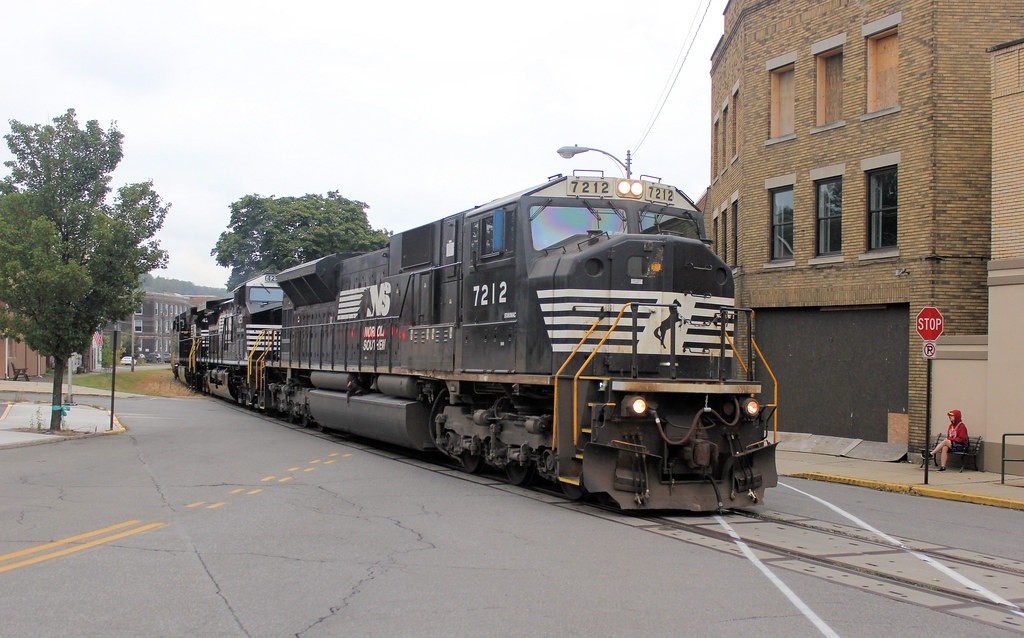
557;144;633;180
109;316;123;429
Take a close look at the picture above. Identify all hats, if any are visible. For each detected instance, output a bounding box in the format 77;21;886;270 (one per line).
947;412;954;416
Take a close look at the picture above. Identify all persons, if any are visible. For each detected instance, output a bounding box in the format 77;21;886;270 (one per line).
921;410;969;473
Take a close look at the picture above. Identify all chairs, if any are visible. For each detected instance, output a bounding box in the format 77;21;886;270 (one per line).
10;362;31;382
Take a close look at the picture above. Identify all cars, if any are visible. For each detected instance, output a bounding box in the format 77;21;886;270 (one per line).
121;356;137;366
148;353;172;363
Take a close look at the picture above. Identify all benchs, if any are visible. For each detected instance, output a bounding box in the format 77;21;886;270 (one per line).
918;432;983;474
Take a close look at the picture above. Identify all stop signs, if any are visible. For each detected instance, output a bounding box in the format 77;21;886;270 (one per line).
915;307;944;341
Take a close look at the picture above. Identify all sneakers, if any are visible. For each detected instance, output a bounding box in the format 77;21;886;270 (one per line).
920;452;934;460
936;465;947;473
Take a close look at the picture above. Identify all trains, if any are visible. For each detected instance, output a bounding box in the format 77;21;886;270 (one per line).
169;169;778;512
